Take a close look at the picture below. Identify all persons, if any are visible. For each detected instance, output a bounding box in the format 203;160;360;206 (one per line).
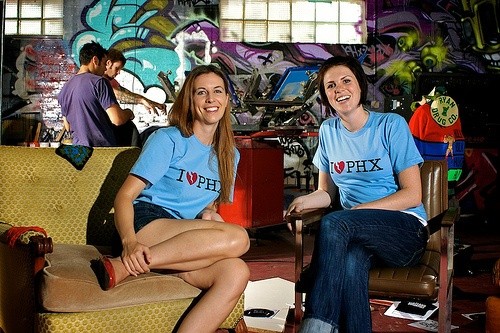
97;65;250;333
102;49;166;116
57;41;135;146
286;57;429;333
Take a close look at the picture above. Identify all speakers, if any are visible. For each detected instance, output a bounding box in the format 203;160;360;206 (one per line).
416;72;500;149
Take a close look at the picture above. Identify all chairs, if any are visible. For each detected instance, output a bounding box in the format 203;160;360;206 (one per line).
290;159;459;333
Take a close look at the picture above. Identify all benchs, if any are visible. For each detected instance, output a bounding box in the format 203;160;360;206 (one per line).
0;145;248;333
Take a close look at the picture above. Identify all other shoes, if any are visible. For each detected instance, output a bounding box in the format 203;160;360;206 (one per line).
97;254;117;289
